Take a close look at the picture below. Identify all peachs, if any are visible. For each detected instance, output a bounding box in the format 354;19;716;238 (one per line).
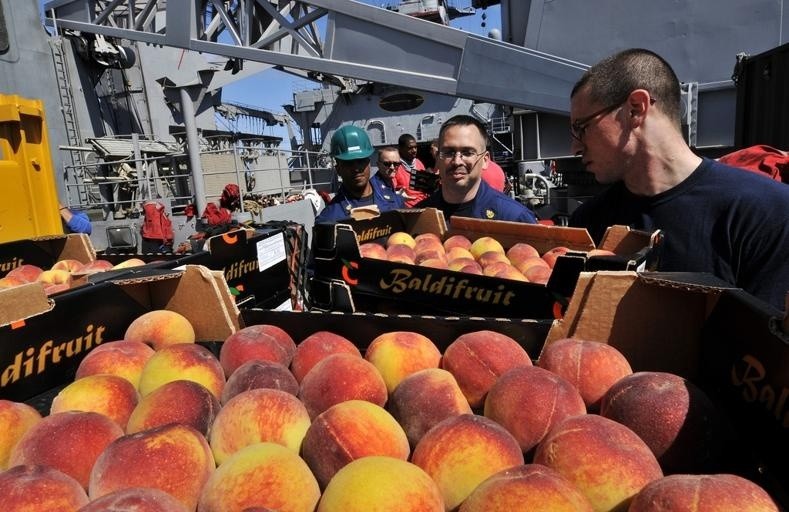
0;258;146;296
0;310;783;512
359;231;618;283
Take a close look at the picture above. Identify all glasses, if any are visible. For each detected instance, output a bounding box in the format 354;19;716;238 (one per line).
435;147;489;161
378;157;402;167
566;95;658;143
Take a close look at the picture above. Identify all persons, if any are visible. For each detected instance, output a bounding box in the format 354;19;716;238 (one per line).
59;205;92;236
371;147;416;208
566;47;788;310
304;124;409;289
425;139;441;174
411;114;538;223
200;184;242;226
481;149;507;194
391;134;429;209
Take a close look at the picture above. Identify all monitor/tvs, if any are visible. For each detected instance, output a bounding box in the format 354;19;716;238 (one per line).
106;225;136;248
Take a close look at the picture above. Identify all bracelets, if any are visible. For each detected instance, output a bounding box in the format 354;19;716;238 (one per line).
60;207;68;211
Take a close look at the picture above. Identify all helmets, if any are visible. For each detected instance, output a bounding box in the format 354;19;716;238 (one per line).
328;123;376;163
